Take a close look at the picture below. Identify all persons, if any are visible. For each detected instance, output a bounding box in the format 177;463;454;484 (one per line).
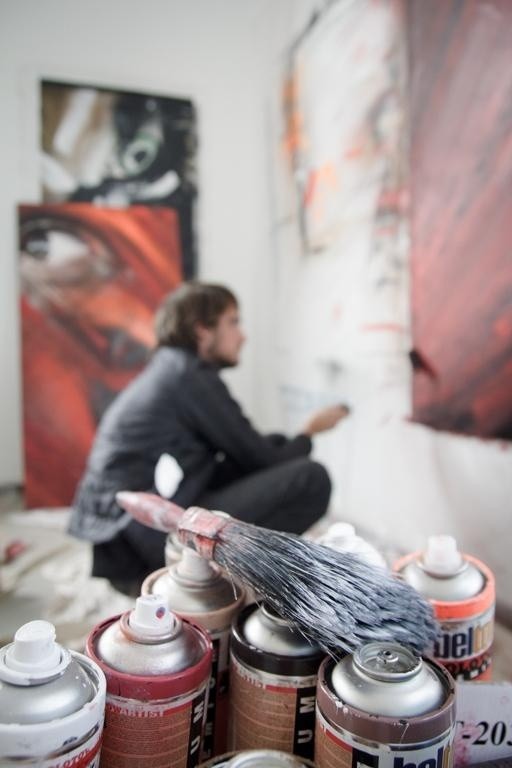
66;282;352;598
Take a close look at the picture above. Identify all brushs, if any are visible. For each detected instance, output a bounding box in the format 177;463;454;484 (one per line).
116;491;443;664
